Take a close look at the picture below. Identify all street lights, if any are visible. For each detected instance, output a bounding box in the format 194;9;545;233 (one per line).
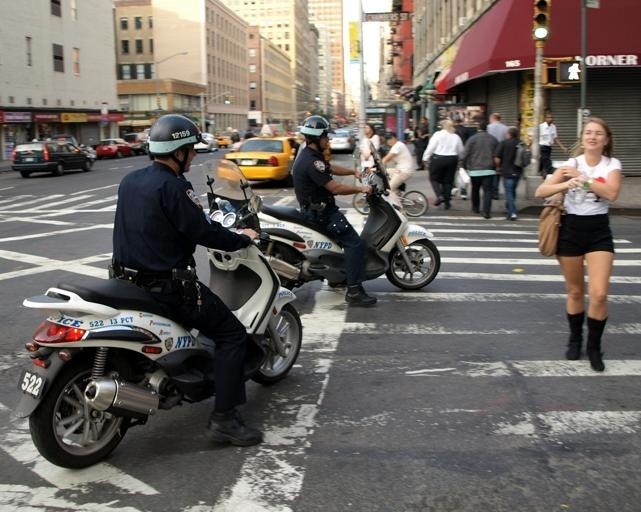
155;51;189;111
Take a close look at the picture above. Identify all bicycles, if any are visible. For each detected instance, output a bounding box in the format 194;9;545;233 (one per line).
351;166;430;217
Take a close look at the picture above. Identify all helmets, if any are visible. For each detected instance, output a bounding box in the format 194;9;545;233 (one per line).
147;114;209;155
300;115;330;136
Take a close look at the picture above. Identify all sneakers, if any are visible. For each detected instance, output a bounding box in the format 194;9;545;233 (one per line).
206;410;261;446
472;193;518;220
434;193;467;209
345;284;376;305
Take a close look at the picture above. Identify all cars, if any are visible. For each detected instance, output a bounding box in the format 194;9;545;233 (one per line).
9;125;243;178
327;127;358;151
218;123;301;190
287;125;304;135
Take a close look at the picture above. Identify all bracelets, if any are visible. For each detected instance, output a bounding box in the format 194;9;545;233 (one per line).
584;178;594;189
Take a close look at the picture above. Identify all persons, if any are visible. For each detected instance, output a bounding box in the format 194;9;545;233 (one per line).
535;117;623;372
359;111;568;221
292;115;378;308
107;113;263;449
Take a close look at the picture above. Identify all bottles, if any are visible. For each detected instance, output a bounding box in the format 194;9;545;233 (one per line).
214;198;243;221
573;169;590;204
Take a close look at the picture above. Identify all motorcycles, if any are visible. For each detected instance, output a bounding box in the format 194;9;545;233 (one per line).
9;158;303;472
256;137;442;291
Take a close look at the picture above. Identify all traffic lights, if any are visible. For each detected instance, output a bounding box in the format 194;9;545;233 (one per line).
156;95;161;105
230;96;235;106
531;0;551;41
557;60;582;83
223;96;229;105
543;61;571;89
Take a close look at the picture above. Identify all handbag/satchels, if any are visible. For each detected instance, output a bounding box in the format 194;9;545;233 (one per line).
514;142;531;168
538;192;564;256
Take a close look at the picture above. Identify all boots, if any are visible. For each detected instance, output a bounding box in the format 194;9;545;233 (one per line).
587;317;608;370
567;312;585;360
